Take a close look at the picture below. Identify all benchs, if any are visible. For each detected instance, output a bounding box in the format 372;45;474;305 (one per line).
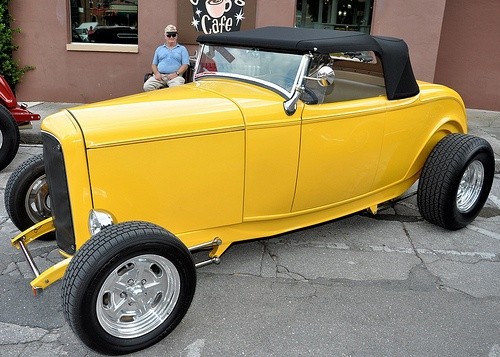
324;71;384;103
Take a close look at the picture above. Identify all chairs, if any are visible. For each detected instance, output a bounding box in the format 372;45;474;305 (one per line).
143;64;193;89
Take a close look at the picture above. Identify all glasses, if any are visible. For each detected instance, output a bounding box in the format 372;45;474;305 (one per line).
166;34;176;37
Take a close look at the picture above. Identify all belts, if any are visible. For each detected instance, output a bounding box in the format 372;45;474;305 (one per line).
160;73;171;74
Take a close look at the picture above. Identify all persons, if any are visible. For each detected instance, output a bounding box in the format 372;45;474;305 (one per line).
143;24;190;92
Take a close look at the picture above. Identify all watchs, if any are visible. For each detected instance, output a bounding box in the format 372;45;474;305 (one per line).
176;71;179;76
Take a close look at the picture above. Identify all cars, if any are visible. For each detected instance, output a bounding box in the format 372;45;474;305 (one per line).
0;74;41;171
2;25;495;357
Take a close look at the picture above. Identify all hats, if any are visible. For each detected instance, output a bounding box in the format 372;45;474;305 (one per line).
164;24;178;33
